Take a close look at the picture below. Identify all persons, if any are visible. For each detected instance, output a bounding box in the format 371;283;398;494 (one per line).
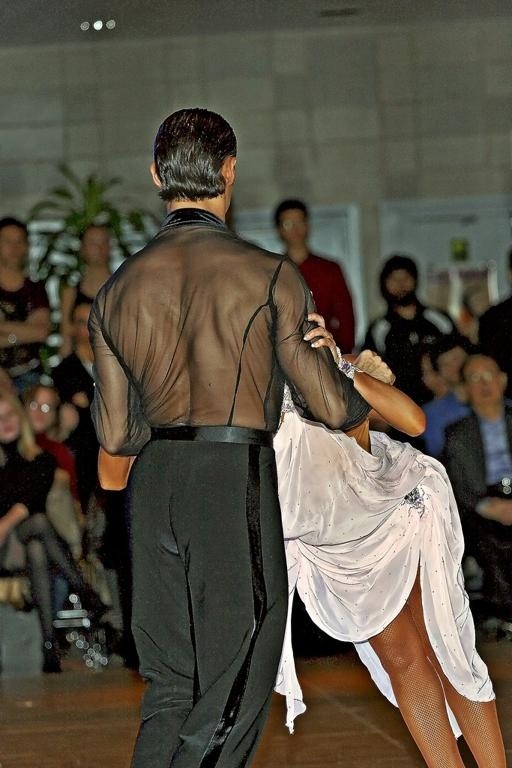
270;309;509;768
86;105;398;767
0;217;144;674
278;200;512;631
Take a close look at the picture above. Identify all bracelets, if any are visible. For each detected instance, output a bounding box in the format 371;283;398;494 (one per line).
337;355;358;381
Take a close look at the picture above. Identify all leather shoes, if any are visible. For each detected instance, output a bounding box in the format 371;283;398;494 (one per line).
77;587;109;616
41;644;62;673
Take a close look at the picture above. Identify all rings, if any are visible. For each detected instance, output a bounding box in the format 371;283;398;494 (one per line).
323;328;328;337
